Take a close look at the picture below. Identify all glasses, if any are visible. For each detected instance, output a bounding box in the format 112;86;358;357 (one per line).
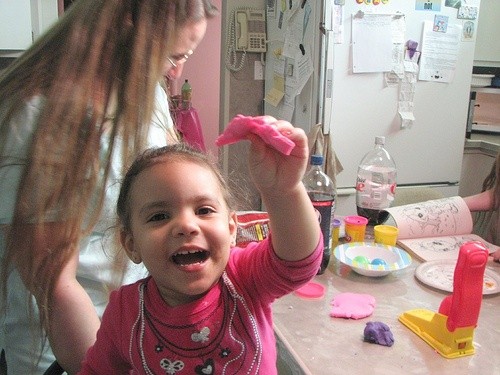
165;49;195;68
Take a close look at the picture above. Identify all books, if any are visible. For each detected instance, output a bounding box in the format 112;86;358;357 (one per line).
379;196;500;262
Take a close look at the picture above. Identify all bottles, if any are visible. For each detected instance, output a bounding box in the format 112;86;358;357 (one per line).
301;154;338;276
356;136;397;240
181;79;193;110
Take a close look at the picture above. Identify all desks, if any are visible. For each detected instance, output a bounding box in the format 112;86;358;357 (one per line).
272;244;500;375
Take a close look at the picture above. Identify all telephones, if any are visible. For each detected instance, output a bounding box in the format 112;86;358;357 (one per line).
234;9;267;53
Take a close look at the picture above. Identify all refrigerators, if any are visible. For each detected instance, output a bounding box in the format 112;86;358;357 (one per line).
263;0;479;218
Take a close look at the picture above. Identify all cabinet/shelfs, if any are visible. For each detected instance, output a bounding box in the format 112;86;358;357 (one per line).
473;0;500;66
0;0;59;56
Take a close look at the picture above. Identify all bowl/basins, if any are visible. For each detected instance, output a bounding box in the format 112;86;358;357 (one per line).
332;241;412;277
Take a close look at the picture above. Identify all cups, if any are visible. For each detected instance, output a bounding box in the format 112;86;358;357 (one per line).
374;225;399;246
343;215;369;243
331;219;342;251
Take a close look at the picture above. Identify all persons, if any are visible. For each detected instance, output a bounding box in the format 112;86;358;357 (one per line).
0;0;218;375
462;150;500;264
78;115;323;375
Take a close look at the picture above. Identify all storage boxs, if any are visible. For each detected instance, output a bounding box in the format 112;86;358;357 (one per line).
472;74;495;89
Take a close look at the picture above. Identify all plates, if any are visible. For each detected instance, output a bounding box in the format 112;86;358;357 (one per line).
414;259;500;296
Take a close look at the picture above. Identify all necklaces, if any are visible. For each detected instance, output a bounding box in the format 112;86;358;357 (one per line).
129;271;263;375
